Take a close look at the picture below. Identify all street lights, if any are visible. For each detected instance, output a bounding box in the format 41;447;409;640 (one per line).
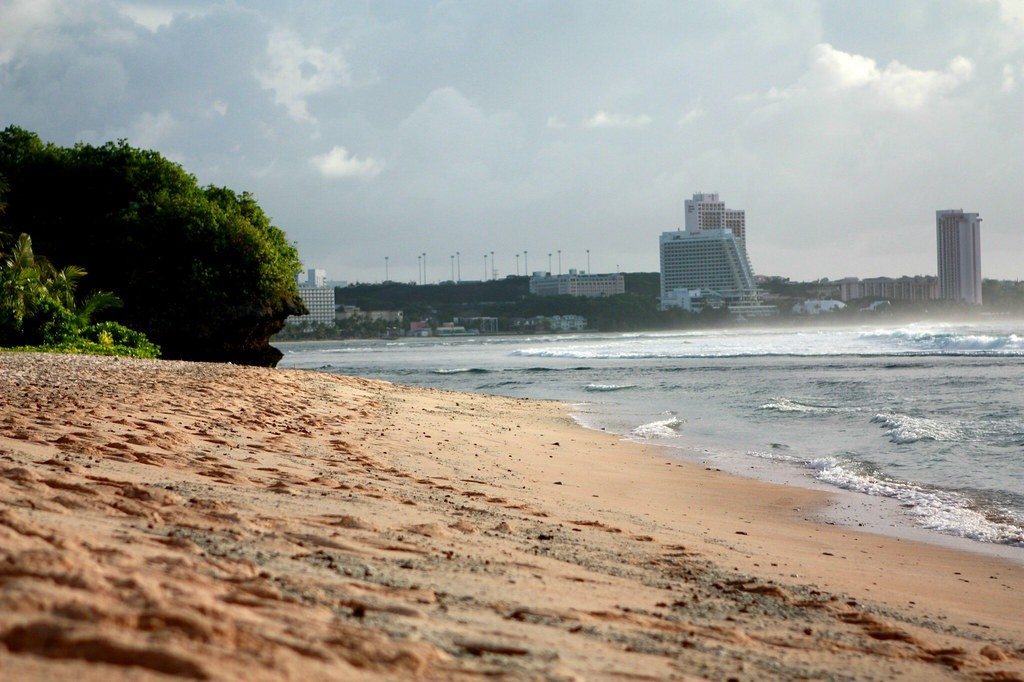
385;248;591;284
